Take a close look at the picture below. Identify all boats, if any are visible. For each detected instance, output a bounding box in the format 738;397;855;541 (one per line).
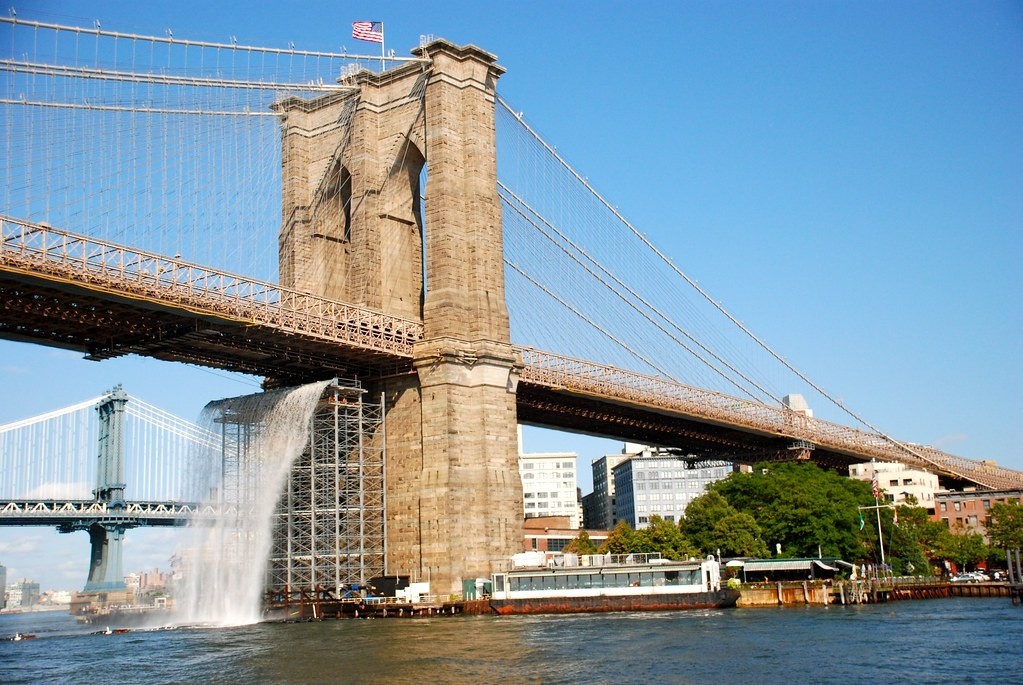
9;632;39;641
725;557;860;606
490;551;742;614
90;626;130;635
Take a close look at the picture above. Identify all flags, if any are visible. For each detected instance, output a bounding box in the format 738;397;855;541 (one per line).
859;514;864;530
871;468;878;498
352;22;382;43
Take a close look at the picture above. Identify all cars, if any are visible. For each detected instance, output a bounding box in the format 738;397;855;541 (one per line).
949;568;1007;583
